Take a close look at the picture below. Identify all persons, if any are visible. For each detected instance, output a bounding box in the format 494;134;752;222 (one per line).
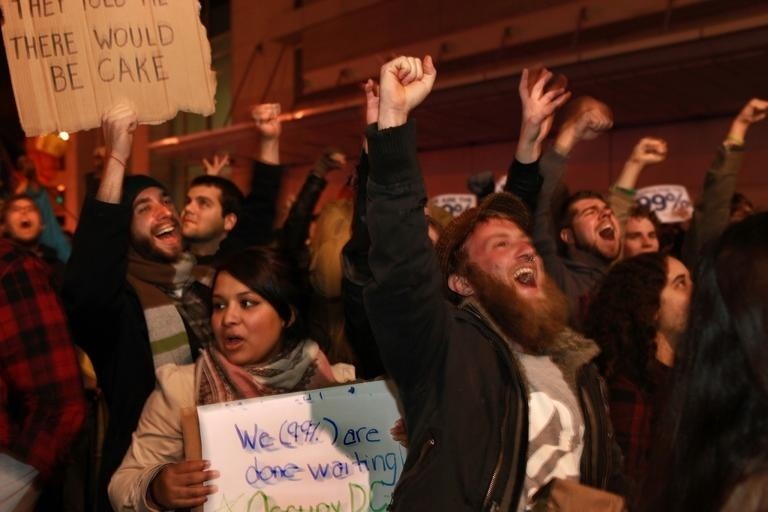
0;55;768;512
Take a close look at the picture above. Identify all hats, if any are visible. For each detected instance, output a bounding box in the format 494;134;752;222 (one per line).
435;191;531;294
110;174;164;233
